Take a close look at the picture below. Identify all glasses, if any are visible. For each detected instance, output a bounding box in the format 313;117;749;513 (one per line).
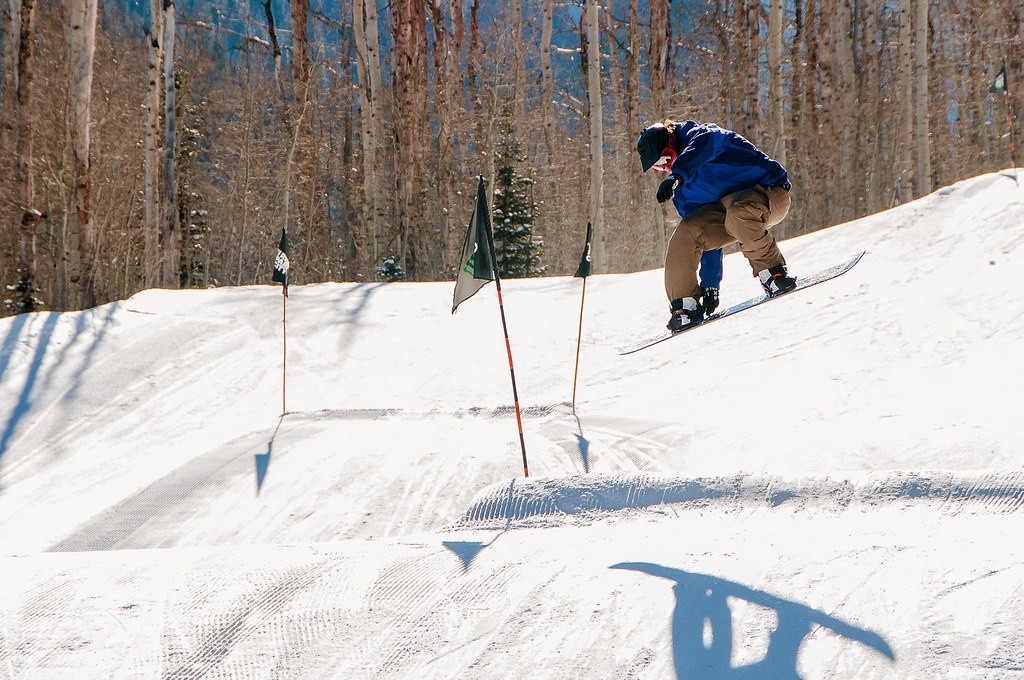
652;152;671;171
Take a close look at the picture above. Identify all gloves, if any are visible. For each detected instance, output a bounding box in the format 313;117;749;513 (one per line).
698;288;719;317
656;173;681;203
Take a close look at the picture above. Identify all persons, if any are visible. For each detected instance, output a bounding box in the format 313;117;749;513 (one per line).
637;121;795;333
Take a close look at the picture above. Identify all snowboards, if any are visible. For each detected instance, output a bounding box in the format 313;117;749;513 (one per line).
617;250;867;355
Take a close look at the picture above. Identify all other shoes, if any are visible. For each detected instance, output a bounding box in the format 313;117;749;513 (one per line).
666;296;703;332
758;267;795;297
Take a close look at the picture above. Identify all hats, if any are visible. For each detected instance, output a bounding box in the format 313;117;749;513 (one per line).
637;122;671;173
